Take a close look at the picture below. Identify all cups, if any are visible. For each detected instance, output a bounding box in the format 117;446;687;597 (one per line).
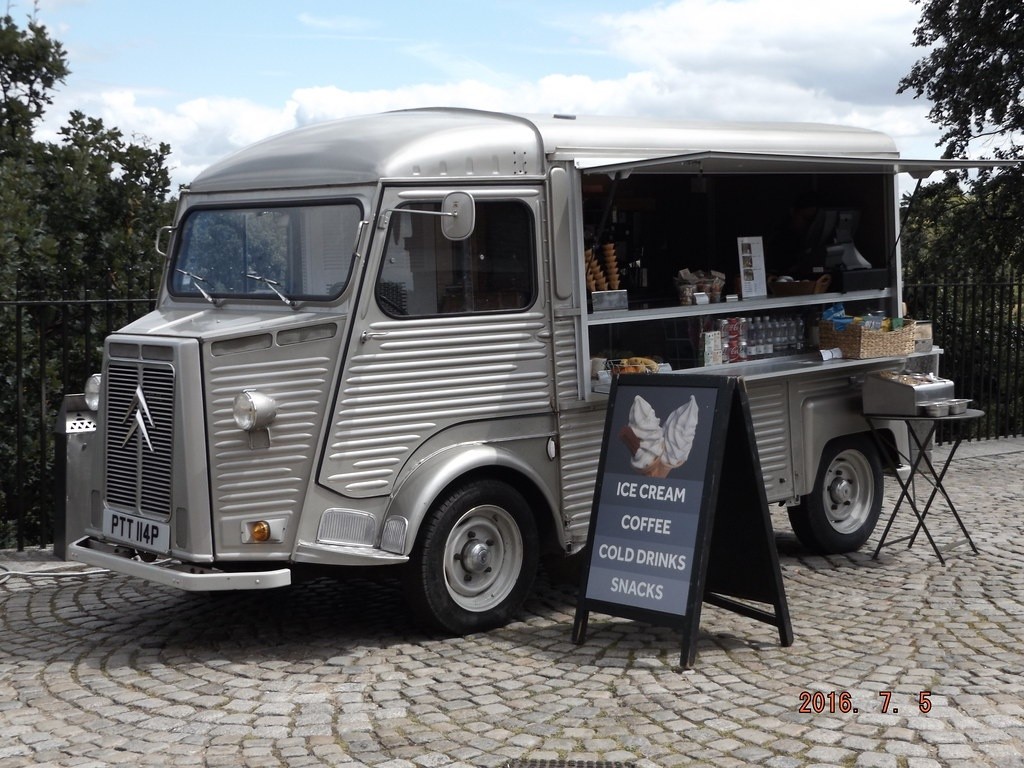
590;358;606;377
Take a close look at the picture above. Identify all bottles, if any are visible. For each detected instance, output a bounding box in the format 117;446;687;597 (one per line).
772;314;804;350
747;318;756;360
754;317;764;359
763;316;773;357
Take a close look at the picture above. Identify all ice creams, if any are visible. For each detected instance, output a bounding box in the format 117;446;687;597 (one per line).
620;394;698;478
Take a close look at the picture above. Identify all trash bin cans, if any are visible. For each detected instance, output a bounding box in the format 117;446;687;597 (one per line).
52;392;97;563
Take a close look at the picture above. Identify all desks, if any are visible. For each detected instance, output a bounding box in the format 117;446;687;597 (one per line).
851;408;986;568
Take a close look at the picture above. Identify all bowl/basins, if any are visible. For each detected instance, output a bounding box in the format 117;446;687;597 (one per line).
680;283;721;306
598;370;619;384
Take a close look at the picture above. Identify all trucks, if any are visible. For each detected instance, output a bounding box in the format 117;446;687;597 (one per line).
54;105;1024;637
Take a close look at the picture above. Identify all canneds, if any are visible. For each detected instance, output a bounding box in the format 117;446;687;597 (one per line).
717;317;748;364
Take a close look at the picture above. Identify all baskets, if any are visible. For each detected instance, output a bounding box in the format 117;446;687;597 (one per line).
819;316;915;359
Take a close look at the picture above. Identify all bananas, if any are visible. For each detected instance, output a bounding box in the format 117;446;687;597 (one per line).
619;357;658;373
